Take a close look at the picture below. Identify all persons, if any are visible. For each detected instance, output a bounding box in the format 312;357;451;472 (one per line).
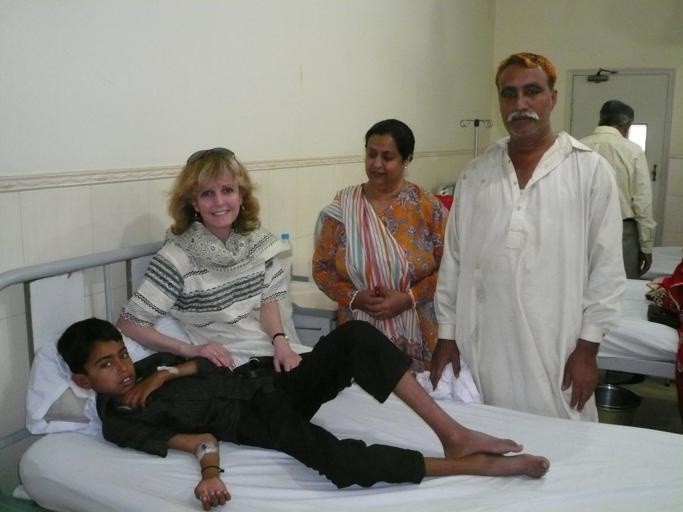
53;315;552;512
312;119;450;375
576;98;658;387
115;147;305;374
427;51;630;426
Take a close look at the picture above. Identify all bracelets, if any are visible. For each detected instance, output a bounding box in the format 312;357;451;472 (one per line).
271;332;288;344
200;465;224;473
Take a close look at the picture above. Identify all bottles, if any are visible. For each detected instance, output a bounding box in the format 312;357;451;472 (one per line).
280;232;293;283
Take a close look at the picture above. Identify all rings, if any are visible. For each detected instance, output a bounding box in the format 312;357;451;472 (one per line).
213;351;218;356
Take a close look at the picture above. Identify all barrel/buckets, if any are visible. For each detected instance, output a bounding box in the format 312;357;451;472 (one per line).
594;382;643;426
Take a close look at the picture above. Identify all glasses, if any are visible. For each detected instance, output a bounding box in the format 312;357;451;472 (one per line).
187;148;234;166
